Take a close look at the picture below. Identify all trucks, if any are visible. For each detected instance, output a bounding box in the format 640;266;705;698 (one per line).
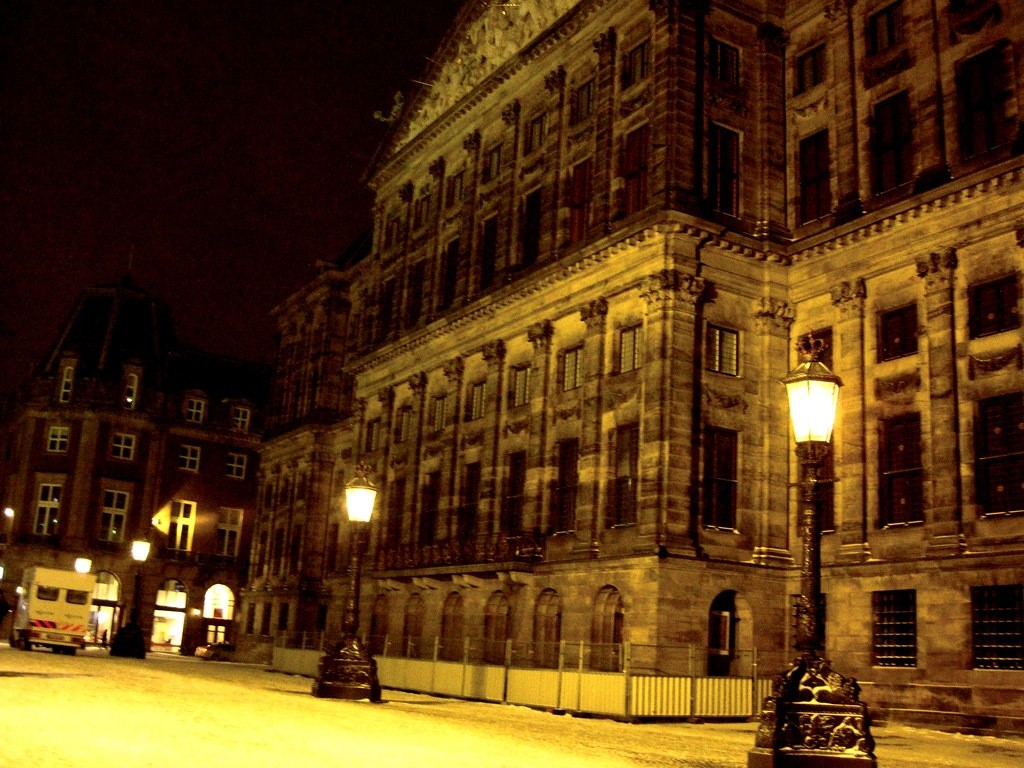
8;564;98;656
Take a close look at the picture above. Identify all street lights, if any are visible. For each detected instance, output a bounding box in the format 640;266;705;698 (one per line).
110;539;154;658
311;459;392;703
744;333;885;768
